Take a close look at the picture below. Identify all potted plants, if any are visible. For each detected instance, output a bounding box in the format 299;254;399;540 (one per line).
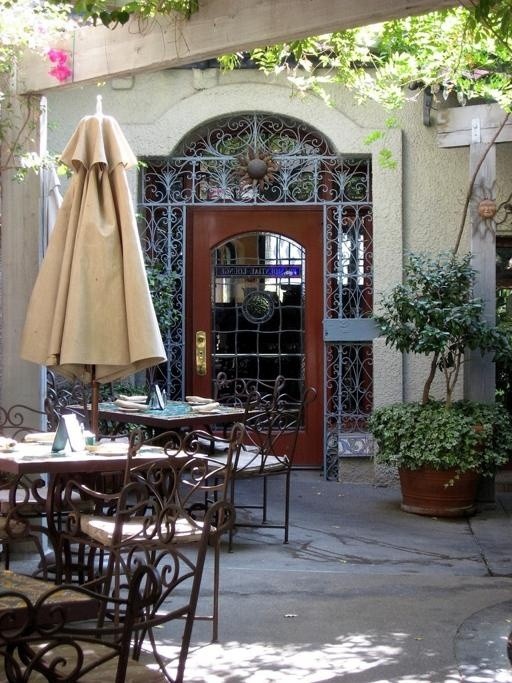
364;249;512;519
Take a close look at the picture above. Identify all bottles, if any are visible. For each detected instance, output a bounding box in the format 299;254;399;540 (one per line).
163;389;168;405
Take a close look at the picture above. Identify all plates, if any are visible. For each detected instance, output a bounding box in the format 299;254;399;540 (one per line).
37;440;54;445
92;442;130;456
187;401;220;414
116;399;147;412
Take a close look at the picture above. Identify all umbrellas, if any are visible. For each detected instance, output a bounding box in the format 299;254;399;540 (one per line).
20;90;168;441
